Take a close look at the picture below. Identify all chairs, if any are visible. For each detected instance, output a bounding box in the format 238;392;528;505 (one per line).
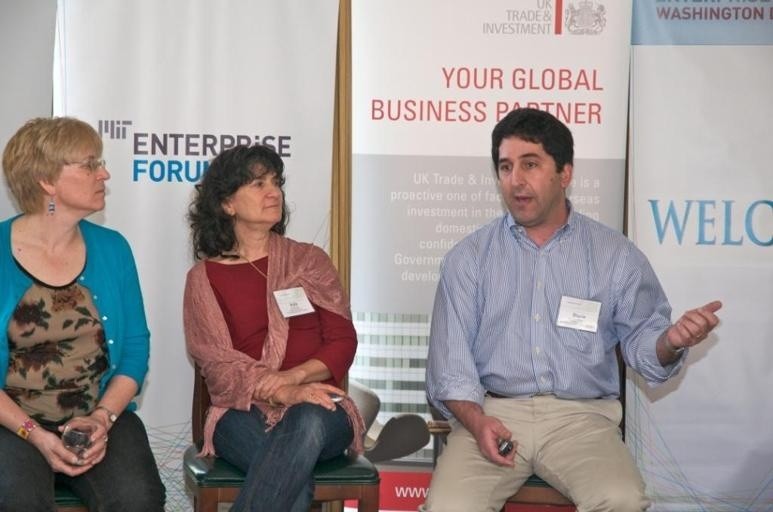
182;359;381;511
438;342;628;512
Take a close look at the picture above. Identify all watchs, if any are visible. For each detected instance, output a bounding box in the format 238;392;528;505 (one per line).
95;404;118;424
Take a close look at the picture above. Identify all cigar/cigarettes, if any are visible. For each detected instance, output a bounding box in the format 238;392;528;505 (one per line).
330;396;347;403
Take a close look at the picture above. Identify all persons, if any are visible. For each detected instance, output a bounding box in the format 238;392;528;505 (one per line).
183;141;360;511
0;114;168;511
417;108;724;512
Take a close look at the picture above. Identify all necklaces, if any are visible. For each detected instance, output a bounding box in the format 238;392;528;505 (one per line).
232;248;273;283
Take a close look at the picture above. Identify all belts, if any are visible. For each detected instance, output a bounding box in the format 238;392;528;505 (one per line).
484;389;604;400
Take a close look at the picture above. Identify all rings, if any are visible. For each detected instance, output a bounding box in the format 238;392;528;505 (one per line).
102;435;109;442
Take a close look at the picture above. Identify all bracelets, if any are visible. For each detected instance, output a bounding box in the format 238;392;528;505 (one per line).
267;395;279;409
16;417;41;439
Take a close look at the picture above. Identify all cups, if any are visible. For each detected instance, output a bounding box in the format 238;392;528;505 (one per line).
62;423;92;455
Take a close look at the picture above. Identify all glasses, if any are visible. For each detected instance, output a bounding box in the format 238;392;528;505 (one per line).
64;158;108;174
